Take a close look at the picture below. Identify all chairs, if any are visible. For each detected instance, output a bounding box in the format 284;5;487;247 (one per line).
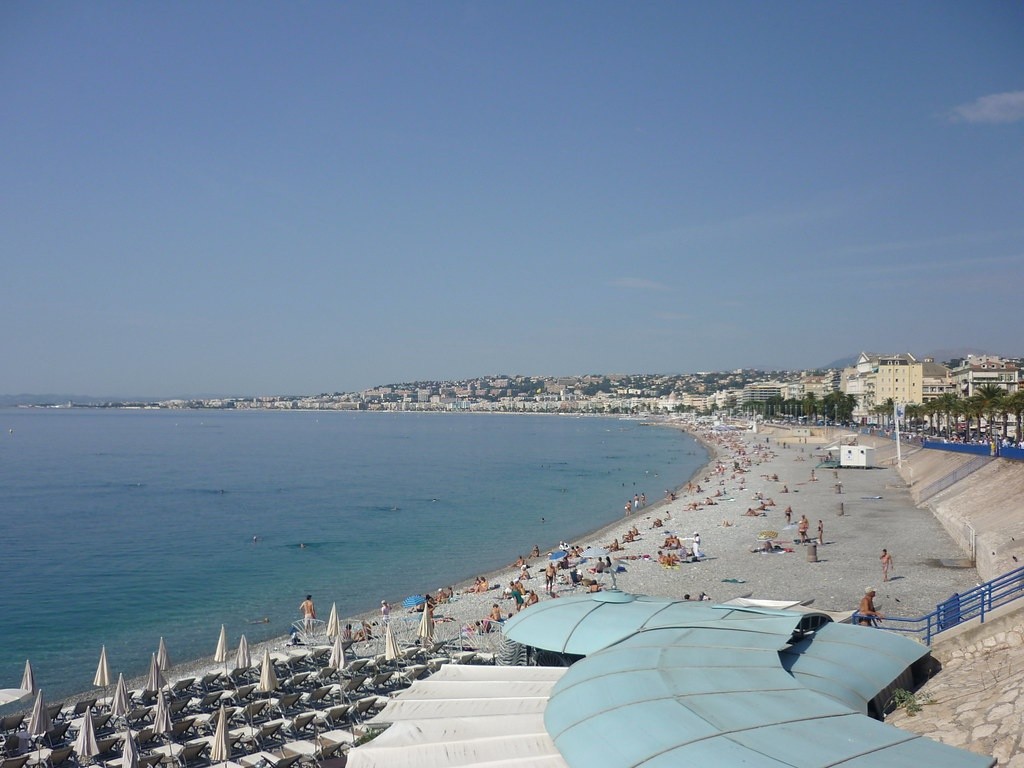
1;635;472;768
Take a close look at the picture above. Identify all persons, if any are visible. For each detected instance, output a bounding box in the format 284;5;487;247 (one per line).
134;418;1024;654
